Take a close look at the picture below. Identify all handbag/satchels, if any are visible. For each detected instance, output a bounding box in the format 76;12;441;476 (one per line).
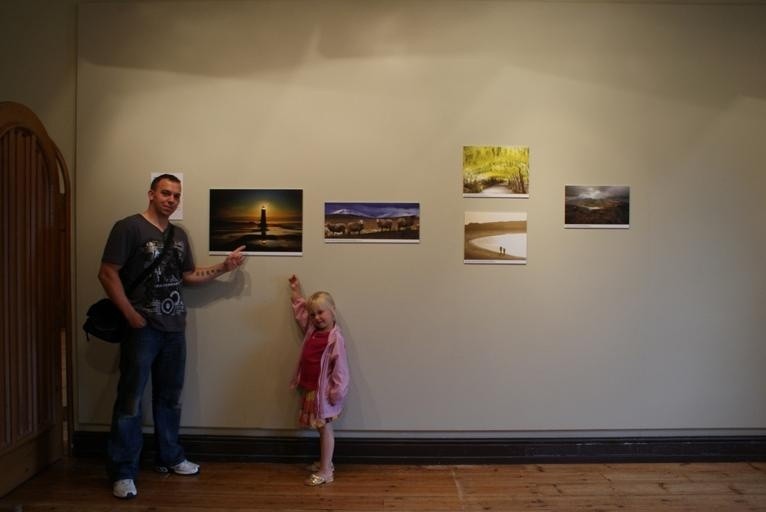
82;298;131;343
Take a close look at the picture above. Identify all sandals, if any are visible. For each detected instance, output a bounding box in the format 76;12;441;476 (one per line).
304;468;335;486
307;459;334;474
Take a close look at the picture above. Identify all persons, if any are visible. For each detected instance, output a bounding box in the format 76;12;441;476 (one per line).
98;175;246;499
288;272;349;486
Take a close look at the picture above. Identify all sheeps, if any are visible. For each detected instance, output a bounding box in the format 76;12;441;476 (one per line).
397;214;417;231
325;222;345;236
347;220;364;236
376;219;393;232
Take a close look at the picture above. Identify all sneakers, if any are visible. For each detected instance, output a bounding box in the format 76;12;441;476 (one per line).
112;478;138;499
153;459;200;475
